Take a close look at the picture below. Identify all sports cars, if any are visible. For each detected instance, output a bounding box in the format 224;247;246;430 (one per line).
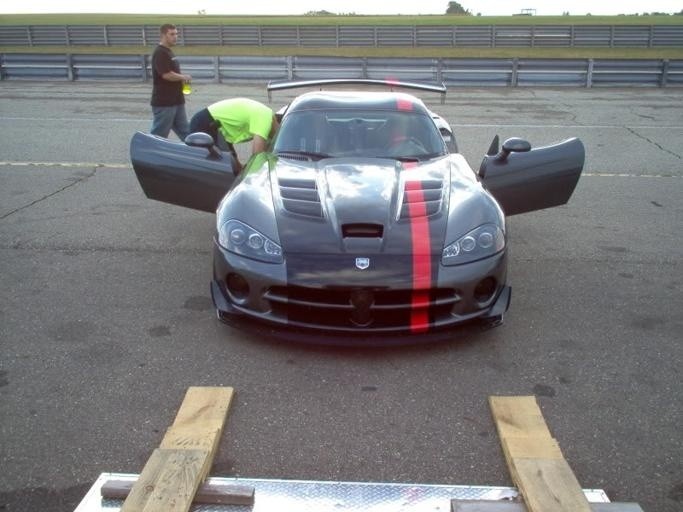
128;78;585;350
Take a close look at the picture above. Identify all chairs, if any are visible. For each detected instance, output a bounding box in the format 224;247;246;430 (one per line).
293;112;335;154
378;118;429;154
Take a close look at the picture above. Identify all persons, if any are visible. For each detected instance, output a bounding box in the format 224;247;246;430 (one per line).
189;97;283;177
150;23;189;144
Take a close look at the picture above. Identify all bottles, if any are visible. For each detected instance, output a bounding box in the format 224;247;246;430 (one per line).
182;80;192;95
